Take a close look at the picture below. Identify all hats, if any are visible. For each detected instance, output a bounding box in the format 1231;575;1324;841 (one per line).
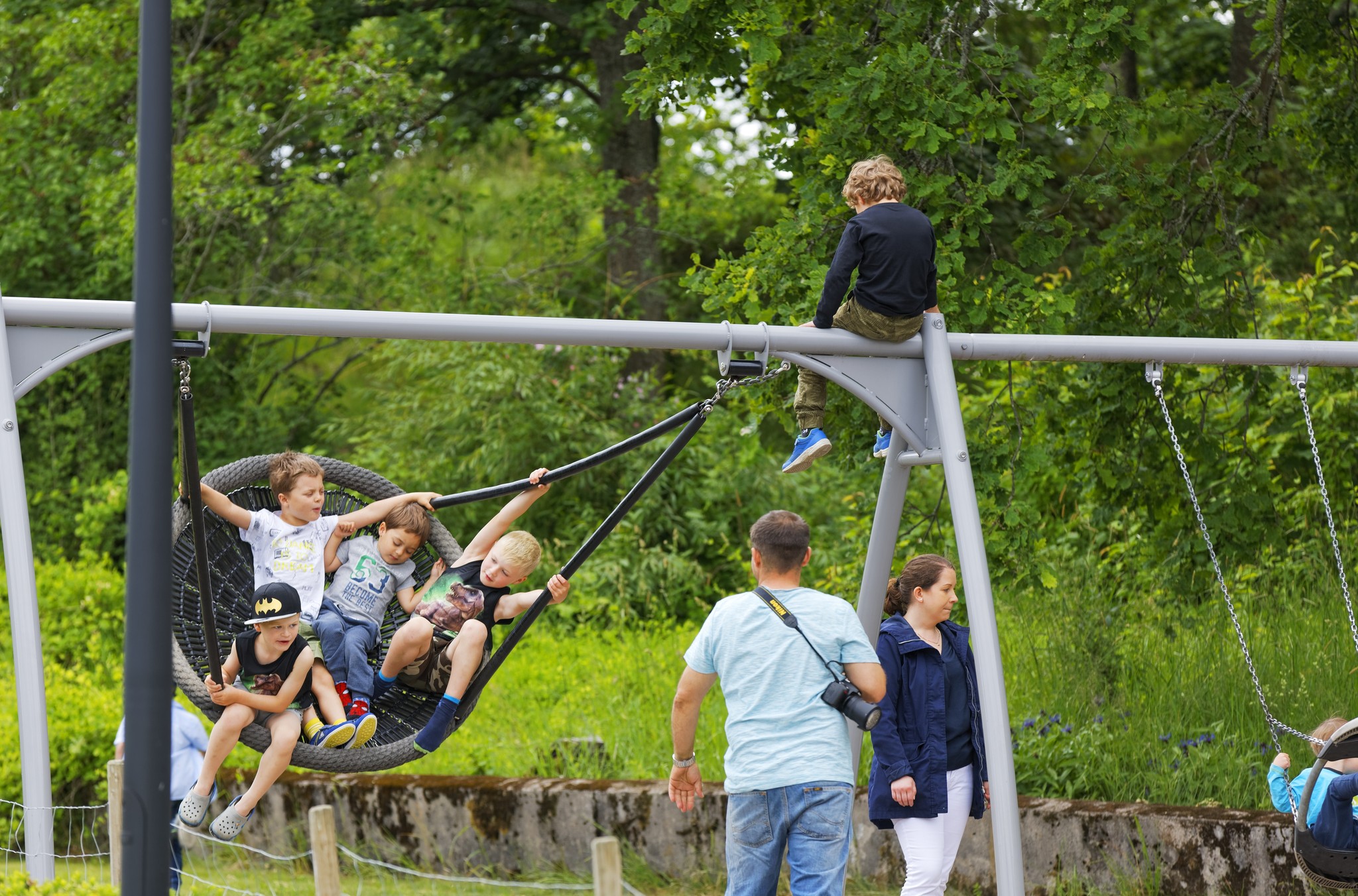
244;582;302;625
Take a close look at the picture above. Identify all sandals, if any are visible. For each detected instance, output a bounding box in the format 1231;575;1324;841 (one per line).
209;794;255;842
178;777;217;828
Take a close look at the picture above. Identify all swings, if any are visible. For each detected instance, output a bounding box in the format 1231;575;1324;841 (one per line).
1139;361;1358;892
152;338;768;776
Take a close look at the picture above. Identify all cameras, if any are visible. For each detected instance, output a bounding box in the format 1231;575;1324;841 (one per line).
823;679;882;731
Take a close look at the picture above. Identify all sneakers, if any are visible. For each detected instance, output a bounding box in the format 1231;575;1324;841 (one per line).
873;430;891;458
782;427;833;473
309;721;357;749
340;712;377;750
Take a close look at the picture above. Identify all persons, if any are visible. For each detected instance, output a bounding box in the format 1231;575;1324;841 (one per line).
178;581;316;842
178;450;443;748
110;682;218;891
781;153;945;474
371;467;570;753
1266;710;1358;852
866;554;994;895
667;509;887;895
312;502;447;721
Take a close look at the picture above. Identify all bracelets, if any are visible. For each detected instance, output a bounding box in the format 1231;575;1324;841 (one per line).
672;752;697;769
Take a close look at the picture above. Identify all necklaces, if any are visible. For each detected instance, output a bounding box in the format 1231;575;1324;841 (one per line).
915;627;941;646
